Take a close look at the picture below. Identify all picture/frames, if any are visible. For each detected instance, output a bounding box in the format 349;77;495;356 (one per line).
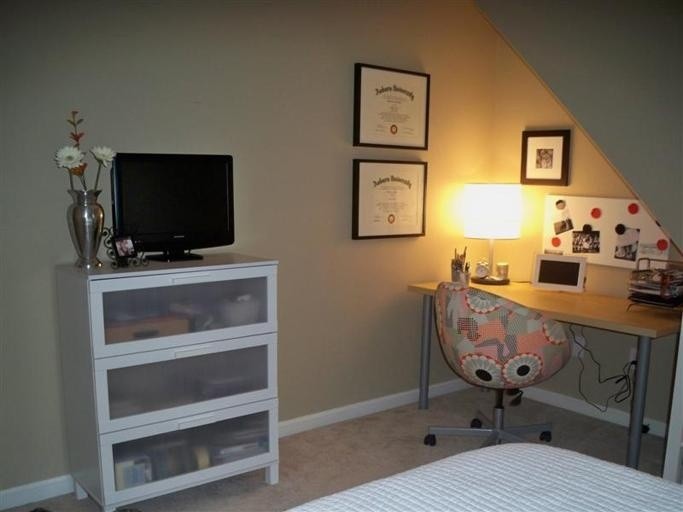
521;130;570;185
354;64;430;238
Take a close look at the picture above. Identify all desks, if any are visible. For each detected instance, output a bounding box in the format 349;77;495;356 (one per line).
407;281;682;469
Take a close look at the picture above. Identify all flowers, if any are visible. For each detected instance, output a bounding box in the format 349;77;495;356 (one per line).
54;110;116;192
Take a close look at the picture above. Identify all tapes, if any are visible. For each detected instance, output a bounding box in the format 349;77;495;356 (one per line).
192;446;210;470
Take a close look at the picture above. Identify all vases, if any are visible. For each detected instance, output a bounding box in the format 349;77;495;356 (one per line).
67;190;104;269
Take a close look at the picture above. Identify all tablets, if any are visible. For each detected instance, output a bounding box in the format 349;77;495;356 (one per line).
533;255;585;293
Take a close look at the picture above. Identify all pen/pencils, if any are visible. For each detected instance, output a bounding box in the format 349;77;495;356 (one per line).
452;246;470;272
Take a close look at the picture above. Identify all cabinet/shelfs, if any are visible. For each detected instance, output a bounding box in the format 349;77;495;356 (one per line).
55;252;278;511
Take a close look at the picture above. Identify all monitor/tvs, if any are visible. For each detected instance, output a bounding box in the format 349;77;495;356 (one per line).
111;152;235;262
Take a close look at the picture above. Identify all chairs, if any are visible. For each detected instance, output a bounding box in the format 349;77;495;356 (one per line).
423;281;574;446
